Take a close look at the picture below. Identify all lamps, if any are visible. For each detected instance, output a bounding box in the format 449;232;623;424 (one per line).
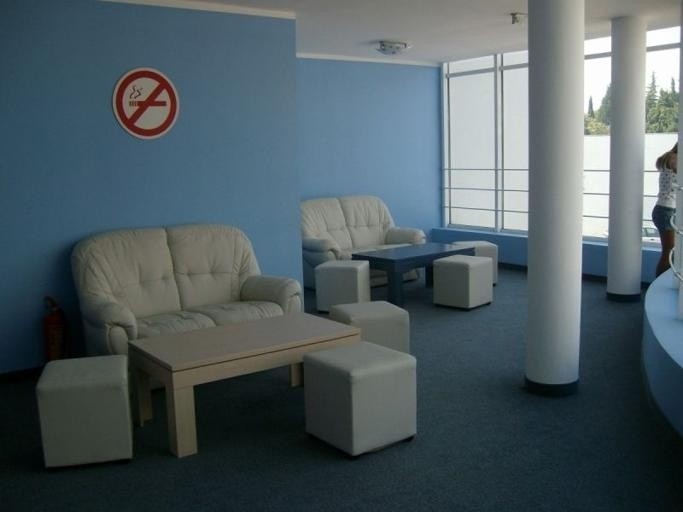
375;41;411;56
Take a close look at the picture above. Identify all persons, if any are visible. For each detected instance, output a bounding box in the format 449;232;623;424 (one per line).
650;141;678;278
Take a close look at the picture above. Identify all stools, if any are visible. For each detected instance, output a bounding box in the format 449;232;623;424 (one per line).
305;339;417;456
36;355;133;468
434;255;494;310
331;301;411;355
453;241;498;284
314;260;370;311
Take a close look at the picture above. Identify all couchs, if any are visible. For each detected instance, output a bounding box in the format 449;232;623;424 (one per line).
301;195;419;290
71;224;304;426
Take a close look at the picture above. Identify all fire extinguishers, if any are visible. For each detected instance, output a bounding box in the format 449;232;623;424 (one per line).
42;296;67;361
